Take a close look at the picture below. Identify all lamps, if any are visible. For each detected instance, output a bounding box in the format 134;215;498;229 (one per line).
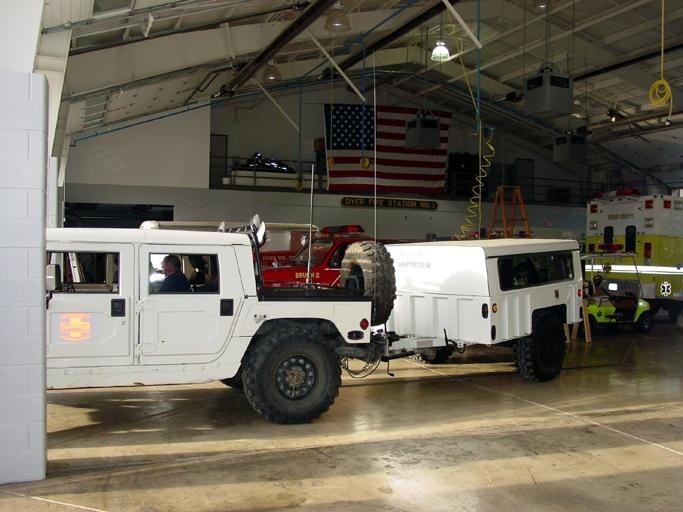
324;0;351;33
430;11;449;62
263;58;281;82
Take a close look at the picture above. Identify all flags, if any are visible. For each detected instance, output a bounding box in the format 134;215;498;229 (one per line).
323;102;455;195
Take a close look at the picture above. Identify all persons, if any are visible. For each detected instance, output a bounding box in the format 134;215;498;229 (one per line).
157;255;193;292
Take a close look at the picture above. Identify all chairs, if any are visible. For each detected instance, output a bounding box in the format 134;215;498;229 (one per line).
189;254;205;285
